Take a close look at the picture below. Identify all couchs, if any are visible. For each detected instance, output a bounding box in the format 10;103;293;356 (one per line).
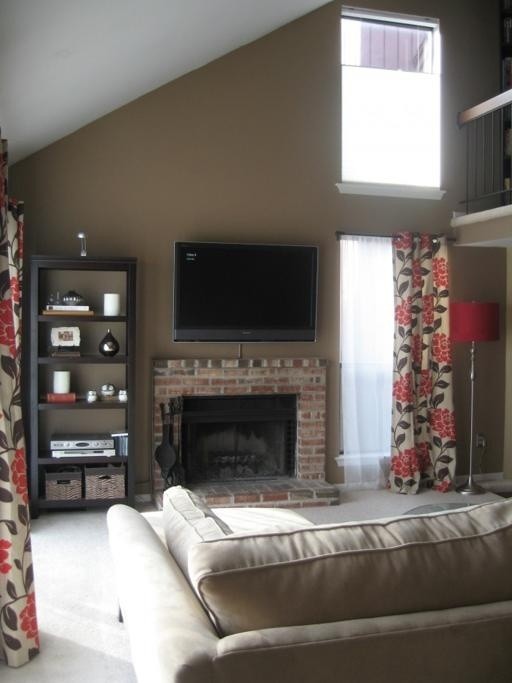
105;485;511;682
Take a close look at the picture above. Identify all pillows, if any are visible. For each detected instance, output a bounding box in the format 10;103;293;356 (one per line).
188;496;511;639
162;485;234;591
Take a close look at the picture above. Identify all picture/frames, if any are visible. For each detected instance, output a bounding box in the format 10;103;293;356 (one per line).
51;327;81;347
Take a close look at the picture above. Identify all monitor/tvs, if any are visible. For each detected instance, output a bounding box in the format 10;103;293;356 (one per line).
173;243;318;342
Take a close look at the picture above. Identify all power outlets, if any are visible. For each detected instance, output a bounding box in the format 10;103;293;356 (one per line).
476;436;486;449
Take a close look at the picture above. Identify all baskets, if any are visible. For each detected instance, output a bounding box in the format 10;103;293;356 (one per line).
84;467;126;500
45;471;83;501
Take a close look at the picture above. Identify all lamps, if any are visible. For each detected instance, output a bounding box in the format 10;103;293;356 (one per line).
449;301;500;495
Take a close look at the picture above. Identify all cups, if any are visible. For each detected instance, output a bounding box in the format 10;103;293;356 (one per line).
103;293;121;317
53;371;70;395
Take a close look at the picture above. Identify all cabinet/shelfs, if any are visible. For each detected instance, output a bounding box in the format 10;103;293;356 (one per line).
28;255;136;520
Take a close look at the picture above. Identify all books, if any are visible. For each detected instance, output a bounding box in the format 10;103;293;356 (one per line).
39;303;94;316
